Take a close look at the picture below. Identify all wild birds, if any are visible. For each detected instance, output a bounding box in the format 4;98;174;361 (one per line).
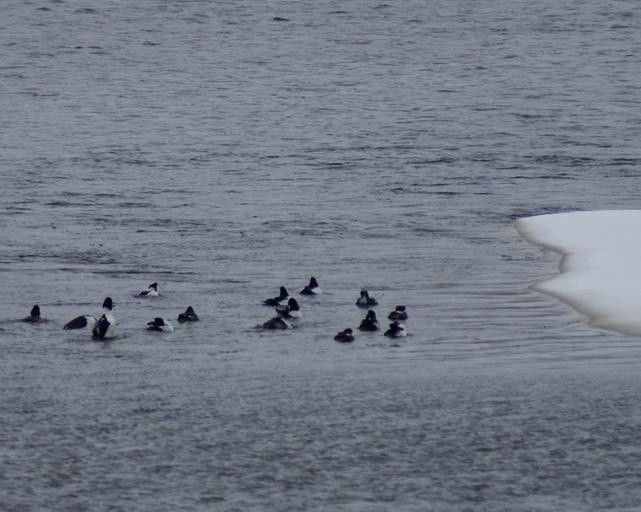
22;305;42;322
334;289;409;343
176;305;199;324
145;317;174;332
262;276;323;330
63;297;117;339
138;282;160;297
